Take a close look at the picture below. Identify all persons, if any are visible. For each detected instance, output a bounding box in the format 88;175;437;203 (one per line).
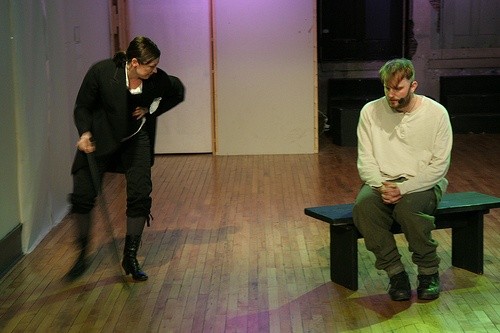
353;59;452;301
63;37;185;282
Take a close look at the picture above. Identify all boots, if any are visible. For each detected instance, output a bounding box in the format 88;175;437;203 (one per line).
66;212;92;282
122;212;154;281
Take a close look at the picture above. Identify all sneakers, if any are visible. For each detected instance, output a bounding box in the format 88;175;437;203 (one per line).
386;271;412;301
416;270;440;300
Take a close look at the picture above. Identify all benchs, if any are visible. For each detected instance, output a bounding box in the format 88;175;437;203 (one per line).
304;191;500;292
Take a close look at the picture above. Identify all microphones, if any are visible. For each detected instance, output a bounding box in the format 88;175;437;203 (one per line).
399;85;411;104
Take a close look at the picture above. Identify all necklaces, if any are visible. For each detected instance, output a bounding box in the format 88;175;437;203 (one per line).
408;96;417;113
